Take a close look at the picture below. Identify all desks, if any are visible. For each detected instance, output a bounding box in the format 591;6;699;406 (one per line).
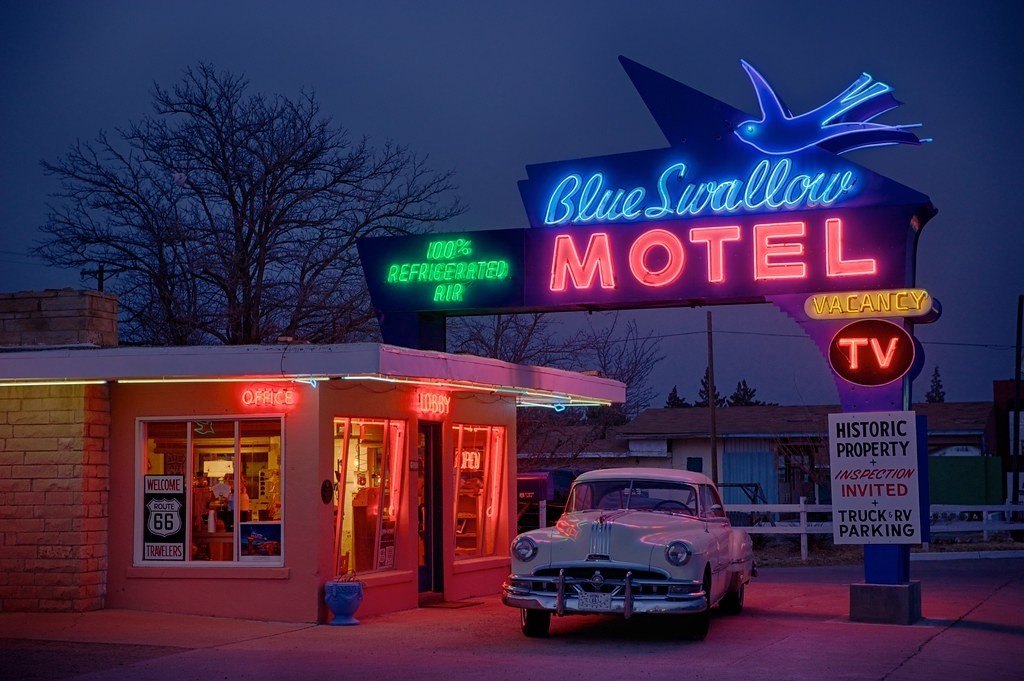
240;520;281;557
193;532;233;562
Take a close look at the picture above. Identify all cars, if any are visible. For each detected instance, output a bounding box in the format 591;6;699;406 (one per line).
501;467;756;643
